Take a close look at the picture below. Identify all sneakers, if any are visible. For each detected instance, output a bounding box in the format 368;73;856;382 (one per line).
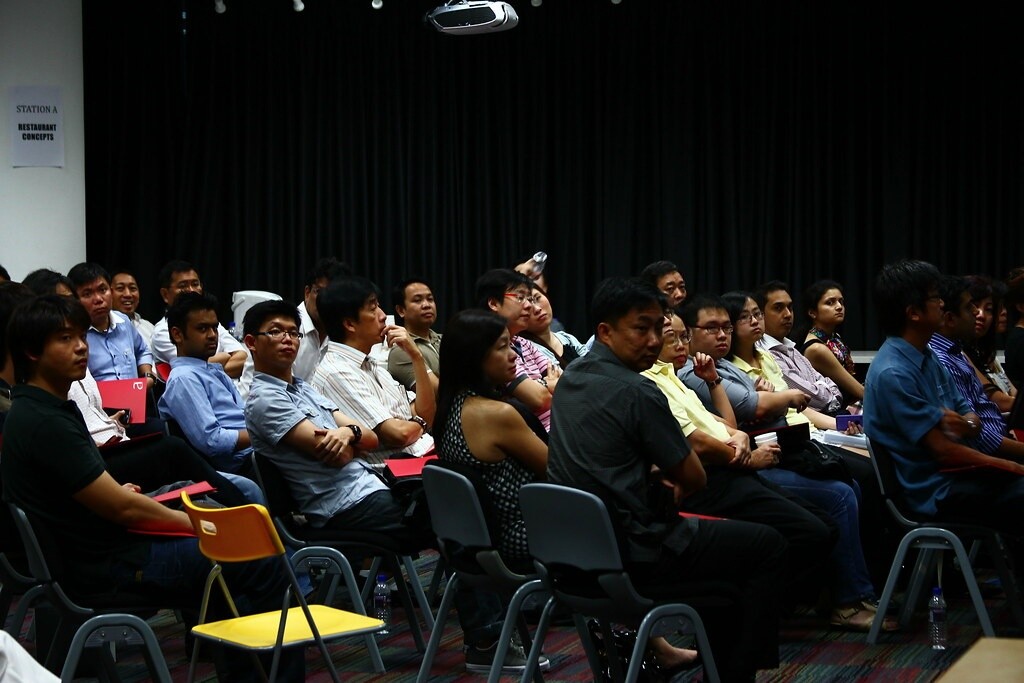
464;638;550;673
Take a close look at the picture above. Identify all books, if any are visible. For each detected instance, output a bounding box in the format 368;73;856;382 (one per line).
824;431;868;447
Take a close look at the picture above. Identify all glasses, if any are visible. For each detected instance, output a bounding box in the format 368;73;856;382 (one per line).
689;324;734;334
916;292;944;304
251;328;304;341
502;293;535;305
736;312;765;323
667;333;693;346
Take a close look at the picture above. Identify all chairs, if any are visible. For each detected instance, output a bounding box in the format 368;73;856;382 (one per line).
864;432;1010;645
0;447;722;683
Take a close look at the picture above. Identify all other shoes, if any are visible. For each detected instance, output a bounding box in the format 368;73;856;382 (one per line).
144;608;177;629
661;650;702;679
949;578;1002;599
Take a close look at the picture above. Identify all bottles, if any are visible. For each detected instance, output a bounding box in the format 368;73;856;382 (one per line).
532;252;548;278
928;587;946;649
372;574;391;634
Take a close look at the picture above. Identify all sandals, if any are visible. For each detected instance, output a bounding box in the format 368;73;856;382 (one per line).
830;608;905;631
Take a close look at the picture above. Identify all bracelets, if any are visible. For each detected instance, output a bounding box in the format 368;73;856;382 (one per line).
968;421;972;433
707;377;723;387
140;373;157;384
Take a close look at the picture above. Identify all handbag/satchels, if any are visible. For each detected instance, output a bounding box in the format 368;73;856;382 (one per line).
589;620;665;683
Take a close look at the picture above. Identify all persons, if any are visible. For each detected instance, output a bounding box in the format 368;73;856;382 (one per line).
1;255;1024;683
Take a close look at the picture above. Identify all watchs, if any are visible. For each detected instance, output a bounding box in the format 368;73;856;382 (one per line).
409;416;426;431
346;425;362;443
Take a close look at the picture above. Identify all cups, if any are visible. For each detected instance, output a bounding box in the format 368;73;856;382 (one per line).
755;431;777;448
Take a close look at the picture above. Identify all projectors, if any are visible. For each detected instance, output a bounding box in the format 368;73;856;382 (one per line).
428;1;519;35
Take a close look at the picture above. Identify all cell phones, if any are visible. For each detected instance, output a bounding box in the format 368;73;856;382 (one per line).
102;407;130;424
836;415;863;431
